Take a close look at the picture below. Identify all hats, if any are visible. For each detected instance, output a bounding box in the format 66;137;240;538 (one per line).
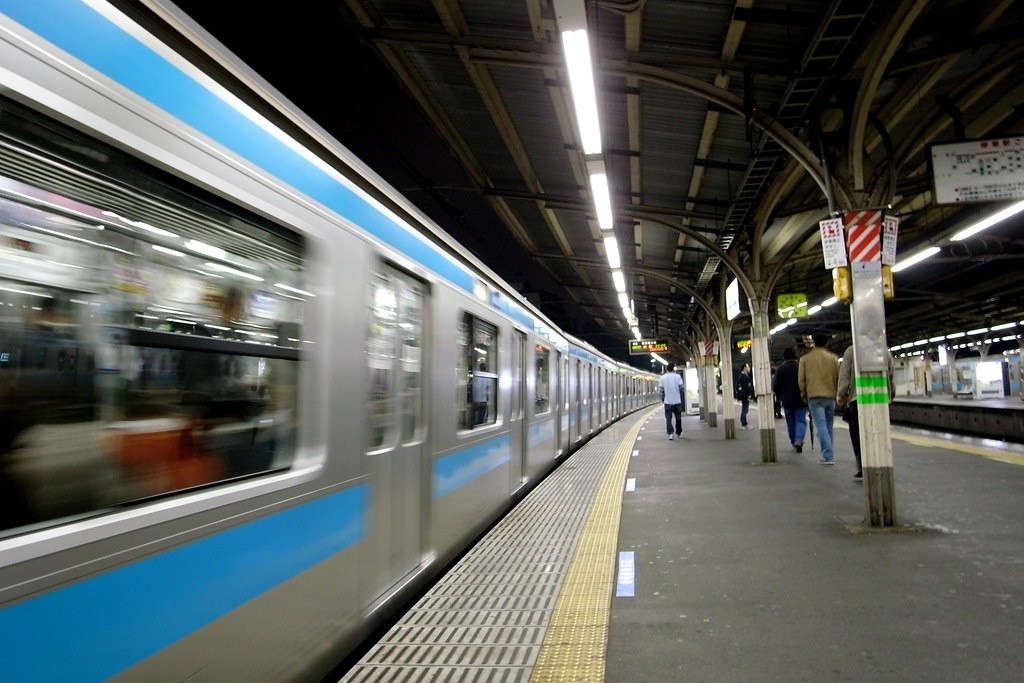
782;347;797;361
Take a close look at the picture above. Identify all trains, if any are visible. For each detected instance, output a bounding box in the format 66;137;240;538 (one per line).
0;1;664;683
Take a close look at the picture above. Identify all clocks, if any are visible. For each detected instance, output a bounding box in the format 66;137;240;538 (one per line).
776;293;808;319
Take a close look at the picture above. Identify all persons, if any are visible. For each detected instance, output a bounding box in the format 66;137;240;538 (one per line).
466;362;491;431
797;333;842;465
659;361;685;441
770;367;783;419
777;346;808;453
735;363;754;430
836;333;896;482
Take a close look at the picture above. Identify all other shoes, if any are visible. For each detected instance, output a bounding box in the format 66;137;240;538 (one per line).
852;470;863;479
741;425;754;430
668;434;673;439
778;415;782;418
817;458;835;464
795;443;802;453
677;432;682;438
